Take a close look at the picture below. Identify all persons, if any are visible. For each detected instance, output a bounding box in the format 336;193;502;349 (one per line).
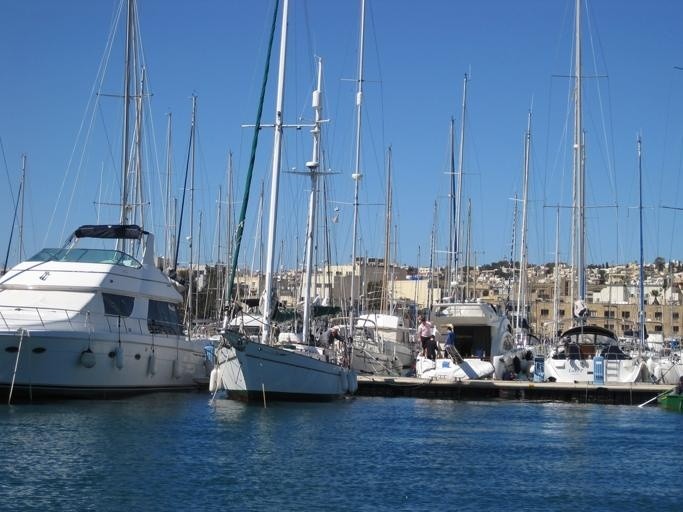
444;327;455;357
318;328;350;350
415;318;434;355
421;335;444;360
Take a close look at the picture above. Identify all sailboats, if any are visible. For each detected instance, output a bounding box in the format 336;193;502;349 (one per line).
202;0;681;391
1;0;220;406
208;0;365;406
